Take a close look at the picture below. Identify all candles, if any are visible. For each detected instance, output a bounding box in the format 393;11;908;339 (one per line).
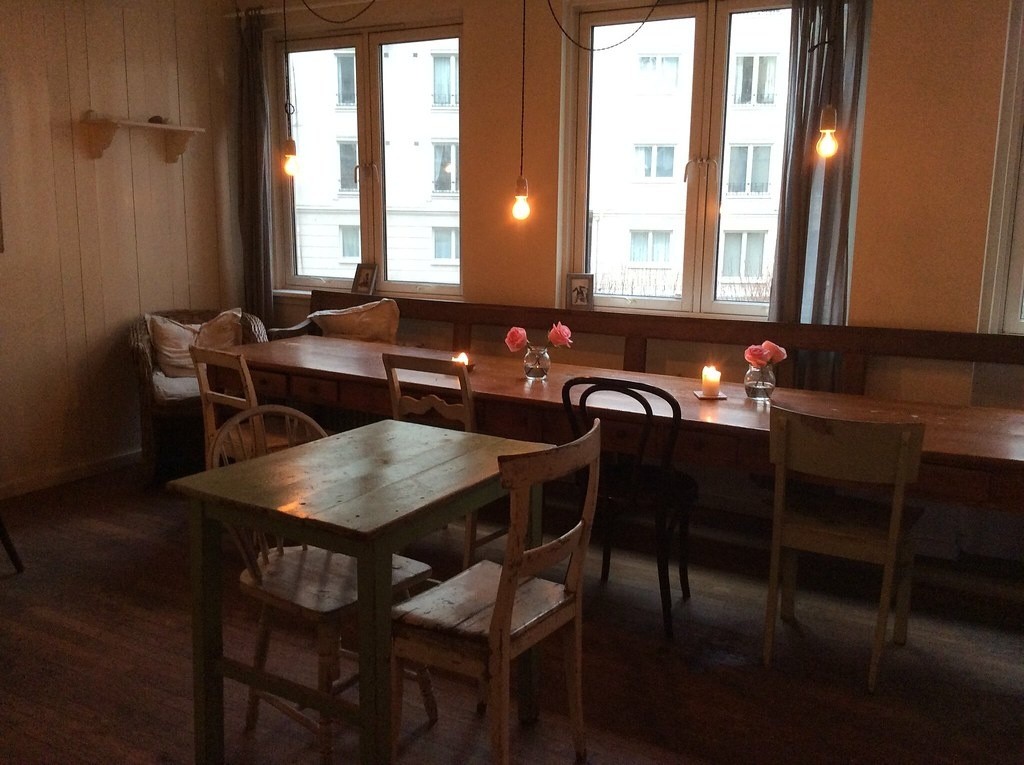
700;364;721;395
452;351;468;367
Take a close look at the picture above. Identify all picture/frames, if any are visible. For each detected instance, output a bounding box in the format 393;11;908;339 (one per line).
351;263;378;294
565;272;596;309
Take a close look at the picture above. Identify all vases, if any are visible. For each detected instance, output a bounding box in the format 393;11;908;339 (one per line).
745;363;776;399
522;346;551;381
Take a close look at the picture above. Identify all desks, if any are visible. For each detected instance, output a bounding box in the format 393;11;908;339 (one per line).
208;334;1023;629
168;420;557;765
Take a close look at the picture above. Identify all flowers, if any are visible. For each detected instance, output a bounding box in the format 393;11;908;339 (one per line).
505;324;534;353
543;320;574;351
743;338;789;399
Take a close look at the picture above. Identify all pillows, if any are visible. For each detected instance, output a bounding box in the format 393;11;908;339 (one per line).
305;299;401;344
144;306;242;377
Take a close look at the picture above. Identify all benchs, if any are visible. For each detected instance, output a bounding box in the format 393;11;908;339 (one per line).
266;289;1024;410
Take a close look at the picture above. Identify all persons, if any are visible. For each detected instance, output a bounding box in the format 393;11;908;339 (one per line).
573;284;587;303
360;273;370;288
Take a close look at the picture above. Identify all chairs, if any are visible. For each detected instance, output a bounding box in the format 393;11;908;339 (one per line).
186;345;337;469
209;402;440;765
560;376;700;635
380;352;508;567
763;404;925;697
128;304;272;478
392;416;600;765
